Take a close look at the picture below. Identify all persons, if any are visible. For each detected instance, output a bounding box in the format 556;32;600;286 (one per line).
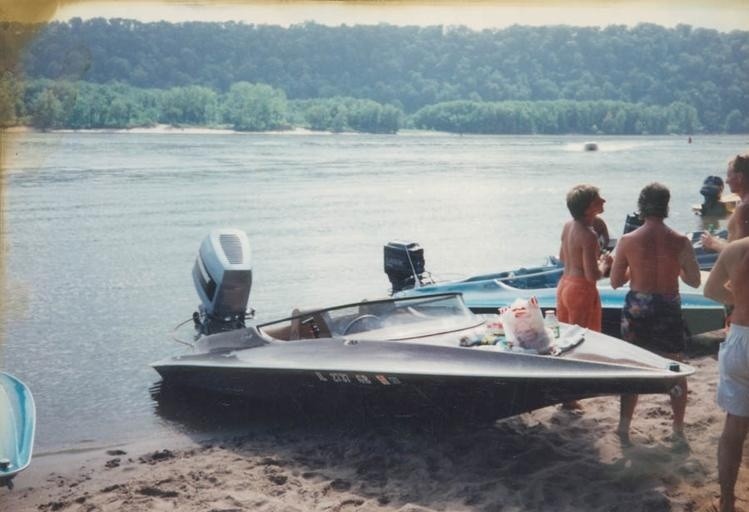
555;185;613;409
695;176;730;217
701;153;749;253
703;236;749;512
609;183;700;434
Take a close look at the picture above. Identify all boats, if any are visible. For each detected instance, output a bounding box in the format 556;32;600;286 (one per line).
146;220;700;420
583;142;598;150
378;239;729;337
692;175;742;216
0;373;36;478
622;209;729;271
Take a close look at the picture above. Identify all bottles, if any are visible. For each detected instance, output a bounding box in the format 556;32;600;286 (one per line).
543;309;560;344
704;224;715;254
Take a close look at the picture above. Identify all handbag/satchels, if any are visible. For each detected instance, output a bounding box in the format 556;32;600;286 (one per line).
497;295;557;355
